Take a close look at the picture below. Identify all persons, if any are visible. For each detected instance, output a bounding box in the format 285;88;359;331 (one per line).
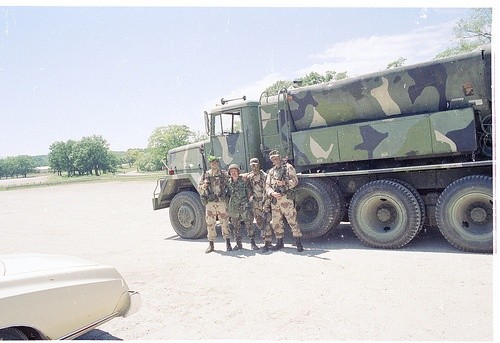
266;149;305;253
239;158;273;253
227;164;259;251
198;155;232;253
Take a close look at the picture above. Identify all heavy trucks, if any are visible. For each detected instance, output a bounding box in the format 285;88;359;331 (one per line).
150;44;494;254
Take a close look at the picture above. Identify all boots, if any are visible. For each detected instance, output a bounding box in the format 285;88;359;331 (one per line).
261;241;271;250
295;238;303;252
233;241;242;249
205;242;215;252
251;239;259;250
272;238;284;250
226;239;232;251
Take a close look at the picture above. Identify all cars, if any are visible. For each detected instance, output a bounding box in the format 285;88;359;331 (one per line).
0;254;135;340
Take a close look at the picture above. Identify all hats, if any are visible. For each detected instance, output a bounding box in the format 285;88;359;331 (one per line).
228;164;241;175
269;150;280;159
249;158;259;165
